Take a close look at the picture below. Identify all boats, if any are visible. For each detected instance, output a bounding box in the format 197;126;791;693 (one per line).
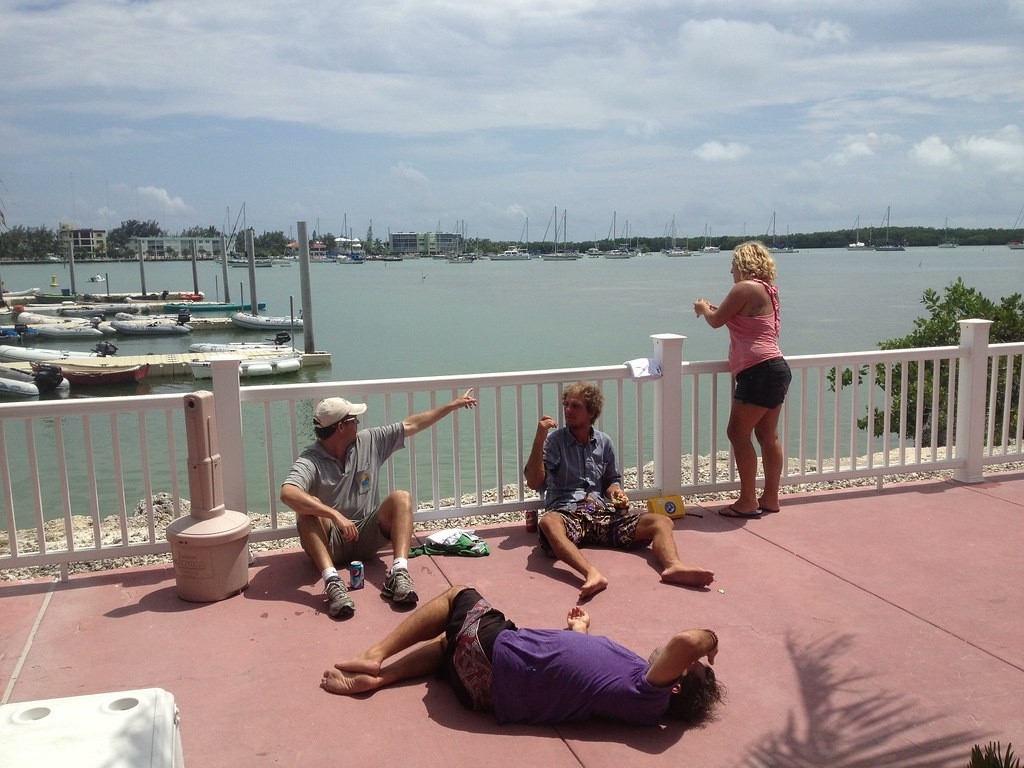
86;277;106;282
29;361;150;383
0;341;118;362
114;309;193;321
188;331;292;353
37;317;117;339
1006;239;1024;249
110;309;193;335
0;290;206;305
0;305;62;319
18;312;106;323
0;323;39;339
232;309;303;330
62;303;151;315
0;364;70;395
182;354;301;379
163;302;266;311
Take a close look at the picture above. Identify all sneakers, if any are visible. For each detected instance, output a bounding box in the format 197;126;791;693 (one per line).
382;568;419;602
322;576;355;616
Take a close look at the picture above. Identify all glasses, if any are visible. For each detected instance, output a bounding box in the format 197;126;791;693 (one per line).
342;416;358;423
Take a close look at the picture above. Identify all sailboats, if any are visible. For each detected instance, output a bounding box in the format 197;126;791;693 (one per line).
848;206;958;251
215;202;720;268
761;211;800;254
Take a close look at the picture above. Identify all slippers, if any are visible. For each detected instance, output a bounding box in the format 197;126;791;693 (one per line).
719;504;762;516
757;498;780;512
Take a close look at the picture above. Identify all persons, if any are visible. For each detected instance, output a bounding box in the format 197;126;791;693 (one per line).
693;241;793;518
280;389;477;616
522;383;714;600
320;584;729;730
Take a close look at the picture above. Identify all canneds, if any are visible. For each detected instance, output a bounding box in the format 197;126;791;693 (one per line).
350;560;364;589
526;510;538;533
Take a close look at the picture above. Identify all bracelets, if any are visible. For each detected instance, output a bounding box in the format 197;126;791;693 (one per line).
702;628;718;653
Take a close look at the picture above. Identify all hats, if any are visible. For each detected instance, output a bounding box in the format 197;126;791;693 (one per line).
314;397;368;428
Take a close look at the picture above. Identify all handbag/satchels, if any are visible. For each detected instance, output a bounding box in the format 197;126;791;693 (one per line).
646;494;703;520
